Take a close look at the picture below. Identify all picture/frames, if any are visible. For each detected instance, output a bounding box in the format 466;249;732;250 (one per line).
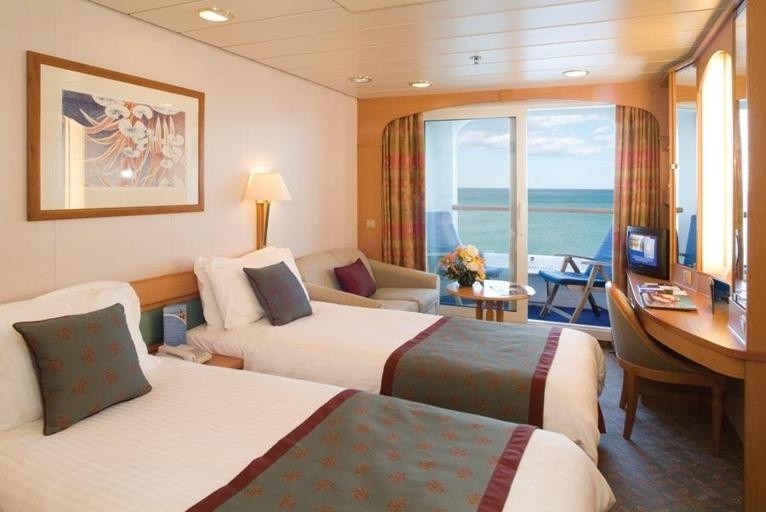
25;50;205;221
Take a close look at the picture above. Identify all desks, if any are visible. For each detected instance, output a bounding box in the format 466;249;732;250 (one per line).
626;271;745;385
445;280;536;322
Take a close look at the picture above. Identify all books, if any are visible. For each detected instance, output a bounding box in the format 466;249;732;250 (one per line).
636;283;698;311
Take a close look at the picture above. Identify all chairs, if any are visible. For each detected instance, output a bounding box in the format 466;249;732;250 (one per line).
425;211;500;305
605;281;725;447
538;225;612;323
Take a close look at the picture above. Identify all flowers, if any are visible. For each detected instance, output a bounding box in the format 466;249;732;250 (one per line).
440;244;486;287
62;90;185;189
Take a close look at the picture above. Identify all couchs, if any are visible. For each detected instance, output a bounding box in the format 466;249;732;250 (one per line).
294;248;440;316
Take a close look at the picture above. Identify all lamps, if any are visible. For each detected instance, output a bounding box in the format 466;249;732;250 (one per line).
241;172;291;248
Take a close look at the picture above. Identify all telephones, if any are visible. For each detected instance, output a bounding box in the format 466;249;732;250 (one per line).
157;343;212;364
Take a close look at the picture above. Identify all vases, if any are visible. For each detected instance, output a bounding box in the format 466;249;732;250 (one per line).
458;279;476;286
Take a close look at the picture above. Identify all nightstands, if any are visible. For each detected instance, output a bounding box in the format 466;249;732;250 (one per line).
148;345;243;370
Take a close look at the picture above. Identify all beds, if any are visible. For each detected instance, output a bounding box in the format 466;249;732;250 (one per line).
0;281;615;512
185;246;605;467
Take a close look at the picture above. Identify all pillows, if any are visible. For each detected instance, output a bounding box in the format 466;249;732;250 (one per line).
13;303;152;436
334;258;376;297
243;261;313;326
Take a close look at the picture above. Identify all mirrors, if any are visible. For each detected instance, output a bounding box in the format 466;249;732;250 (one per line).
674;62;697;271
731;0;747;311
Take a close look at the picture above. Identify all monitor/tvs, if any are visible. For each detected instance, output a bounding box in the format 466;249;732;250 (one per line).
625;224;670;281
